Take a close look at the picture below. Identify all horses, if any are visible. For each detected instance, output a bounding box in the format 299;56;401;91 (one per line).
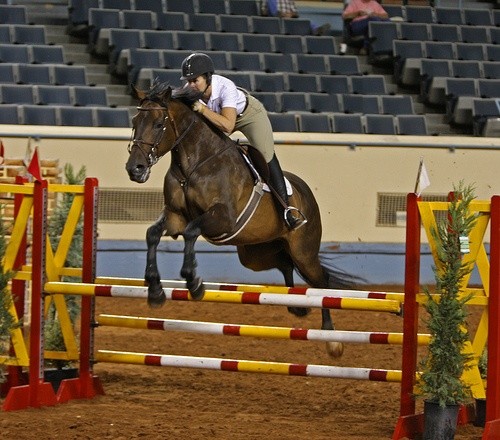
126;83;345;360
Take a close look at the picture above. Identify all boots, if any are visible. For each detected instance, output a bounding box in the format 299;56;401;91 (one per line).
263;154;300;225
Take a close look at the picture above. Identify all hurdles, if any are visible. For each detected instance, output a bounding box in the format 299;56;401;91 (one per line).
1;176;500;437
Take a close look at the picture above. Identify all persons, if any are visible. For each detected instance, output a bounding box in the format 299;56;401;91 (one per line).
342;0;389;36
180;53;302;228
267;0;298;18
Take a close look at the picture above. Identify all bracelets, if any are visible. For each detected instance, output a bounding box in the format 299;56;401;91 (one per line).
201;105;205;113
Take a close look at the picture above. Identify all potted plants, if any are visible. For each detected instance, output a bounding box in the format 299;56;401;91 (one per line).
419;180;482;440
45;162;87;392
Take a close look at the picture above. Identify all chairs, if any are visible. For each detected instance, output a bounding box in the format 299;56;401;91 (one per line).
1;0;500;137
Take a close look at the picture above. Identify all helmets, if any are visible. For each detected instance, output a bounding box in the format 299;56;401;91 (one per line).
179;52;216;82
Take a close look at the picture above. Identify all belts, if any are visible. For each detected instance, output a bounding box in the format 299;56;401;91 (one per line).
236;88;249;120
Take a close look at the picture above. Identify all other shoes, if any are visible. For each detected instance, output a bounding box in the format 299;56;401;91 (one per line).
358;48;367;55
